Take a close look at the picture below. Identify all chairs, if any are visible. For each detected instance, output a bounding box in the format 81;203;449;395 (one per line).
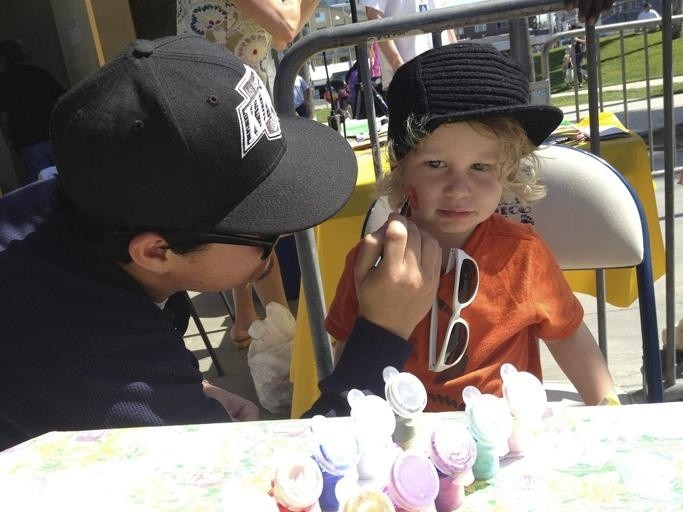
359;147;665;403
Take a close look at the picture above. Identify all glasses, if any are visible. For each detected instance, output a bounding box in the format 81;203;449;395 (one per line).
427;247;479;373
175;230;293;261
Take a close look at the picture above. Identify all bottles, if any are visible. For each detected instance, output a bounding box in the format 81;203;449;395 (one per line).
269;361;549;512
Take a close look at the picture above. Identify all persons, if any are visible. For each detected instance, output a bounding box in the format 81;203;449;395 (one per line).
174;1;325;351
322;40;624;412
0;39;69;187
357;0;456;118
367;37;388;118
560;24;589;90
291;74;312;121
633;3;664;34
0;32;444;452
345;60;367;120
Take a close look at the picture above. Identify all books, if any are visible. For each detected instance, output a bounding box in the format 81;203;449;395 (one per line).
540;118;586;149
578;125;633;142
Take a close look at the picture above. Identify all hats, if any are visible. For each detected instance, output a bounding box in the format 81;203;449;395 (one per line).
47;33;357;233
385;42;563;160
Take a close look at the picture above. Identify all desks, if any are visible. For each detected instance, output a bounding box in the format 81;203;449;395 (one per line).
0;398;683;512
288;110;665;420
